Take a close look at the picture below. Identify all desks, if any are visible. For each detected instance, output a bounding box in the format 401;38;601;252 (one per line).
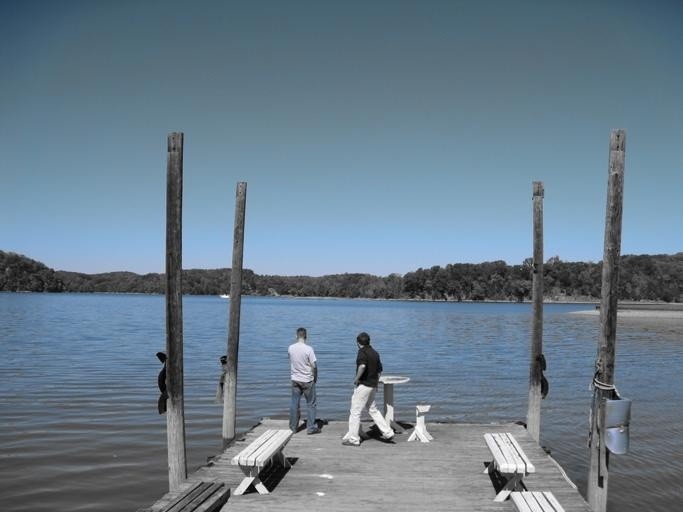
368;376;410;433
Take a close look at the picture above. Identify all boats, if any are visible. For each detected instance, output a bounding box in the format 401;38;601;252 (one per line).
219;294;229;299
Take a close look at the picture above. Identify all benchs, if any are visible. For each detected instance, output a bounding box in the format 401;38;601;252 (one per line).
158;481;231;512
510;492;566;512
484;433;535;501
231;429;293;496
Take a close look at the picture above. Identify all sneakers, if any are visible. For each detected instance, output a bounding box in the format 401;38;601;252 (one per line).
342;439;358;446
307;428;321;434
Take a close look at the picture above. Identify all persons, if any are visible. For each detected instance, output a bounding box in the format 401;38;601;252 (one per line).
288;328;320;435
343;332;394;446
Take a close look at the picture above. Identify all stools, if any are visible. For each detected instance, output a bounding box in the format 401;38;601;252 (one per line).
408;404;433;442
